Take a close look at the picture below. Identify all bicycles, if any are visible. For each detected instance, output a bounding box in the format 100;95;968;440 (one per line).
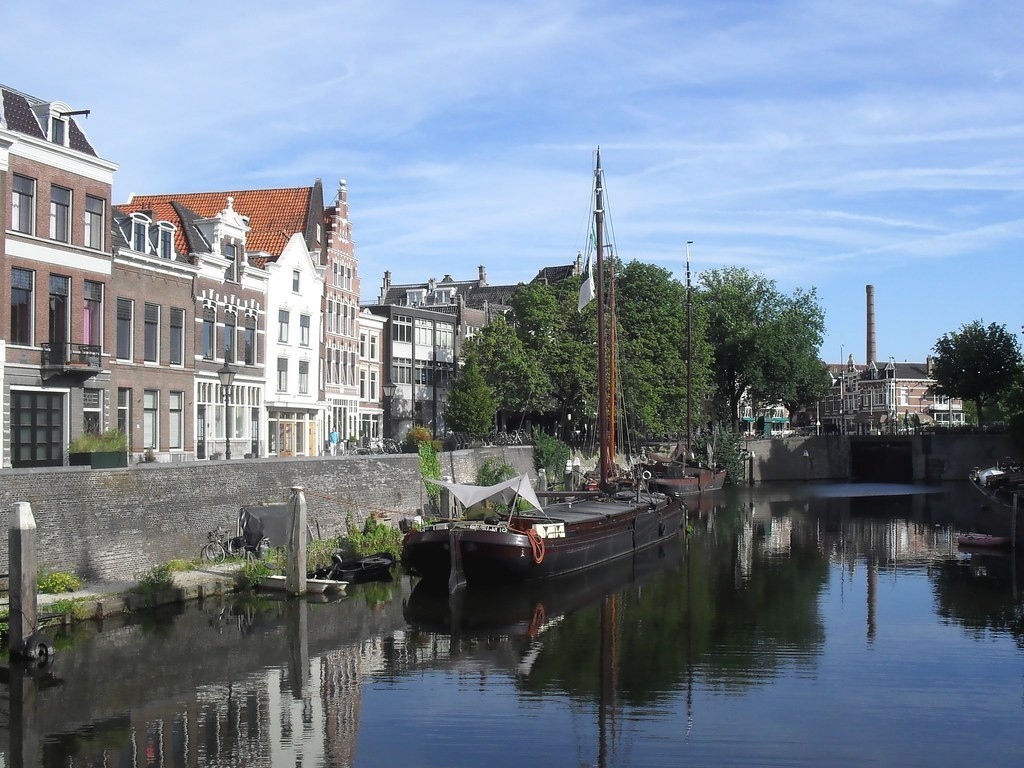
478;423;533;445
349;438;403;454
202;526;246;563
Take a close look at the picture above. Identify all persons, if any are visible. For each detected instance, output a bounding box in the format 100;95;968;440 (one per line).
328;427;340;457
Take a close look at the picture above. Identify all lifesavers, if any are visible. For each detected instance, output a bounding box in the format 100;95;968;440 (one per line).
642;470;651;480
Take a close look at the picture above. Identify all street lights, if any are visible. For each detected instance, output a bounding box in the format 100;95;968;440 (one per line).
217;362;238;461
440;389;450;439
381;379;397;439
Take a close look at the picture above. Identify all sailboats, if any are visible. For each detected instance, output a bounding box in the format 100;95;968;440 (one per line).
397;141;687;592
578;249;645;494
631;240;729;495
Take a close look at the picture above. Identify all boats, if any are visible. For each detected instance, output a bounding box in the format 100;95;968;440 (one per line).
245;573;348;594
969;456;1024;523
958;531;1009;547
316;551;395;583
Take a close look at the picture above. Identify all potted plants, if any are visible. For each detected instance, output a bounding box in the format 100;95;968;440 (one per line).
67;429;129;470
359;430;364;448
347;436;357;450
